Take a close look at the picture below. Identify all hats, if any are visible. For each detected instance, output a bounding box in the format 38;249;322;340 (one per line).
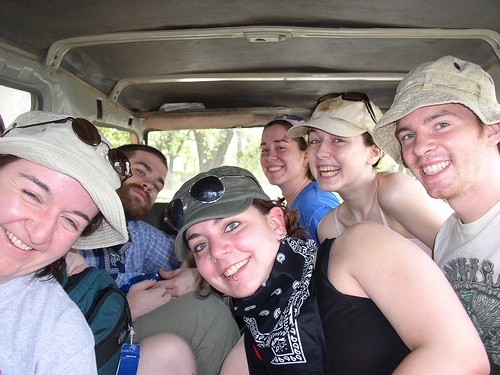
166;166;271;262
0;110;128;251
372;55;500;168
287;96;384;139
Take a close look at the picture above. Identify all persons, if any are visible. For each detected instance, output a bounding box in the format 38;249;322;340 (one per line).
0;111;242;375
287;91;455;261
372;55;500;365
260;114;340;246
165;166;500;375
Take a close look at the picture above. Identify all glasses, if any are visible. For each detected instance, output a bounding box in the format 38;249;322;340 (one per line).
163;174;261;230
317;92;378;124
274;113;306;121
1;116;134;184
